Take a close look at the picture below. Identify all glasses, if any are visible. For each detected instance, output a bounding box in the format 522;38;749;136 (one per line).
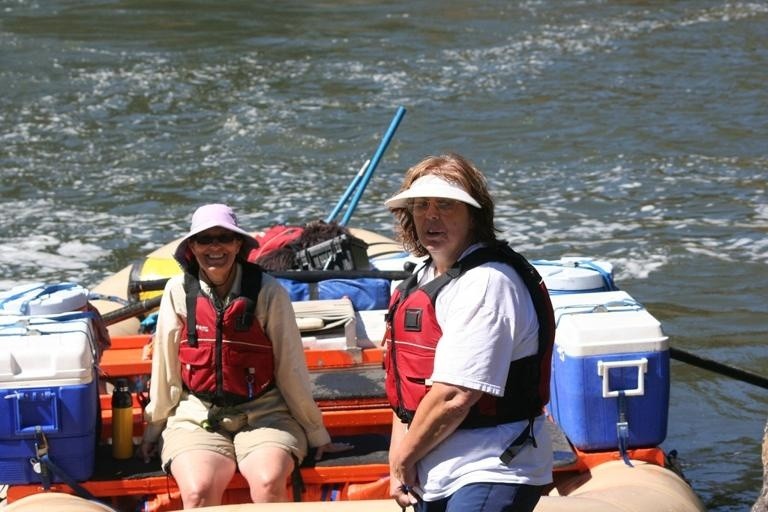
404;200;468;215
194;233;235;245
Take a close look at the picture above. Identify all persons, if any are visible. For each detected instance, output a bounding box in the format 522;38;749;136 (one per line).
372;152;557;512
139;202;357;509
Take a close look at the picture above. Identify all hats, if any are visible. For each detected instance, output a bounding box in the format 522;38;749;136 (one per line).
383;175;483;209
172;203;260;273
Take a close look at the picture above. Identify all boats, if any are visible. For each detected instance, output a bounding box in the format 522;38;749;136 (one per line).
4;225;706;511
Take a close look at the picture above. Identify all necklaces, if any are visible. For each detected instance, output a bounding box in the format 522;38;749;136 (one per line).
202;264;235;288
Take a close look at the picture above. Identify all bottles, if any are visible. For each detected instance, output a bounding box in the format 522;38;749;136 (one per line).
110;376;134;461
